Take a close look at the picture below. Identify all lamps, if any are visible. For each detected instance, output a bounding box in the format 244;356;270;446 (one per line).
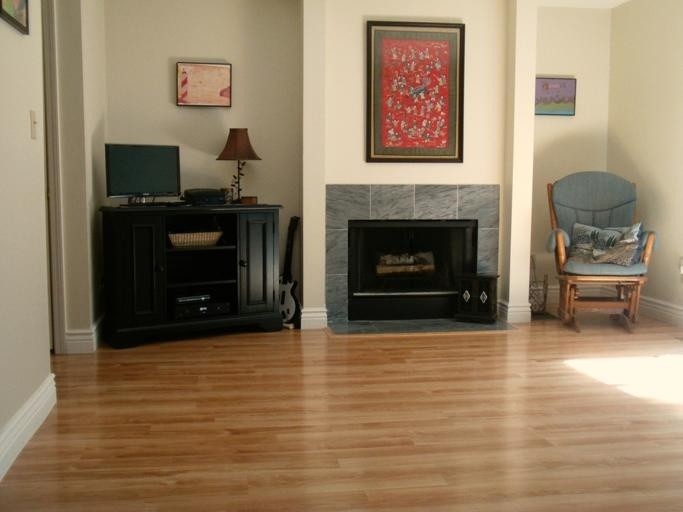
215;127;261;203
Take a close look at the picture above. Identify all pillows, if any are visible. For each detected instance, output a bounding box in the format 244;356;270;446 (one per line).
567;216;643;270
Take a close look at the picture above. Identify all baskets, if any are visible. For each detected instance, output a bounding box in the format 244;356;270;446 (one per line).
164;230;228;247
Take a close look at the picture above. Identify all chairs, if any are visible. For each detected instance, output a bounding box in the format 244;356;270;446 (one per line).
543;170;655;334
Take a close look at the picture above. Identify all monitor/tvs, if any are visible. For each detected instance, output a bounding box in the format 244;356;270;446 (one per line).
105;144;181;209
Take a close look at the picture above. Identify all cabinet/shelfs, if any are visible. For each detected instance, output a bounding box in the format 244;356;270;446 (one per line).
98;204;285;350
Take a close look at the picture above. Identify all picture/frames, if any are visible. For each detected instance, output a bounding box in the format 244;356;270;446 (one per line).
364;19;464;163
0;0;28;34
175;61;231;107
534;77;576;116
